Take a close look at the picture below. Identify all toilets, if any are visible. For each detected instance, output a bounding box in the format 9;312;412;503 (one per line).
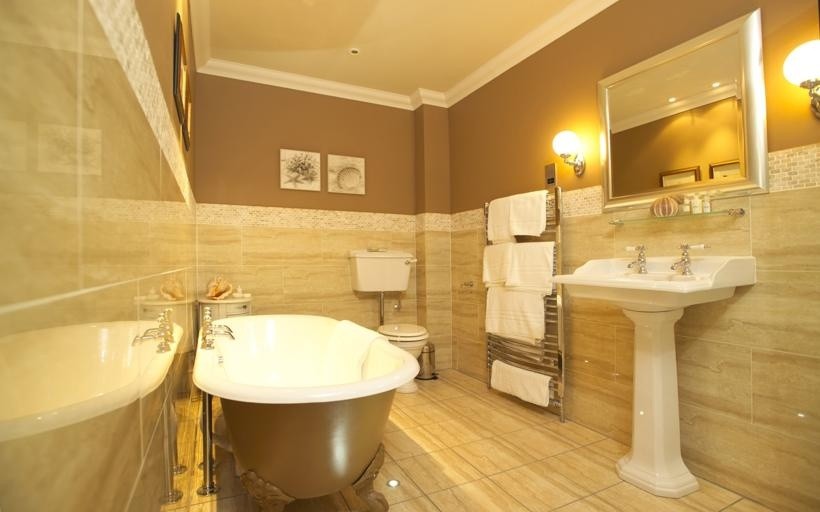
349;252;429;393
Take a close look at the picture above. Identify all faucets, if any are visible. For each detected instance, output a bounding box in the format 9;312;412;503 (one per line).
626;245;648;273
670;244;711;276
201;307;236;349
131;308;174;354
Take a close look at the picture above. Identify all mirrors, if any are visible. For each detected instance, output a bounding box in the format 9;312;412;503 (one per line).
596;6;769;215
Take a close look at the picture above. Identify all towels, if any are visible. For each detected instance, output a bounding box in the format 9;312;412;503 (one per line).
482;189;555;362
490;358;554;409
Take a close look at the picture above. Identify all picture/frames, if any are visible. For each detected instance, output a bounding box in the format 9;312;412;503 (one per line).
182;80;192;152
709;159;740;180
658;165;700;189
172;12;189;126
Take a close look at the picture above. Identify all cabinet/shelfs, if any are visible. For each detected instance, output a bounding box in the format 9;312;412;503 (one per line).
196;293;253;337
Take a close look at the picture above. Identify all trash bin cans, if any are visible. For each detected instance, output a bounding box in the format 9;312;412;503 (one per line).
415;343;439;380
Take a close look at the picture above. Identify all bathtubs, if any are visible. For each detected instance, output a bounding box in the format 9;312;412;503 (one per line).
191;313;421;512
0;321;184;512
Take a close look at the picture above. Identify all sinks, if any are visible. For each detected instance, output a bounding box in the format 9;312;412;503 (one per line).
551;257;757;311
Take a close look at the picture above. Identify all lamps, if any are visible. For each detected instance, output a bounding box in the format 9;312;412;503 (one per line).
551;131;585;178
782;38;820;120
